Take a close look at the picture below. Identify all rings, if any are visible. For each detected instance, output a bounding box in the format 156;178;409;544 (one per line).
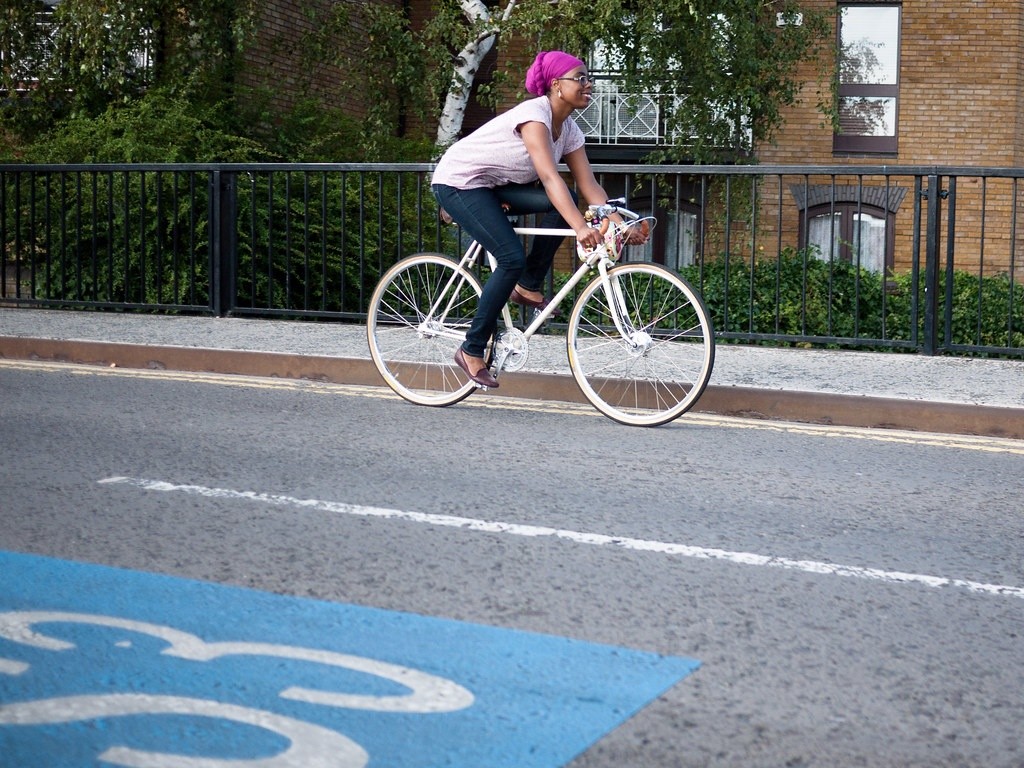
586;243;592;248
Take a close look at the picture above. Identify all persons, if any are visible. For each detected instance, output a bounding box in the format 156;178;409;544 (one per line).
431;50;649;388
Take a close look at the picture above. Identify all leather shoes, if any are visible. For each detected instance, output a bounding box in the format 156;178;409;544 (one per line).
454;346;499;388
509;288;562;315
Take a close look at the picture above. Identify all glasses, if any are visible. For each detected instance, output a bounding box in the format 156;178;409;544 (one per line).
556;76;595;87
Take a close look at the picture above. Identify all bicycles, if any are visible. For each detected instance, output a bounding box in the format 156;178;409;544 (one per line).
366;196;717;428
